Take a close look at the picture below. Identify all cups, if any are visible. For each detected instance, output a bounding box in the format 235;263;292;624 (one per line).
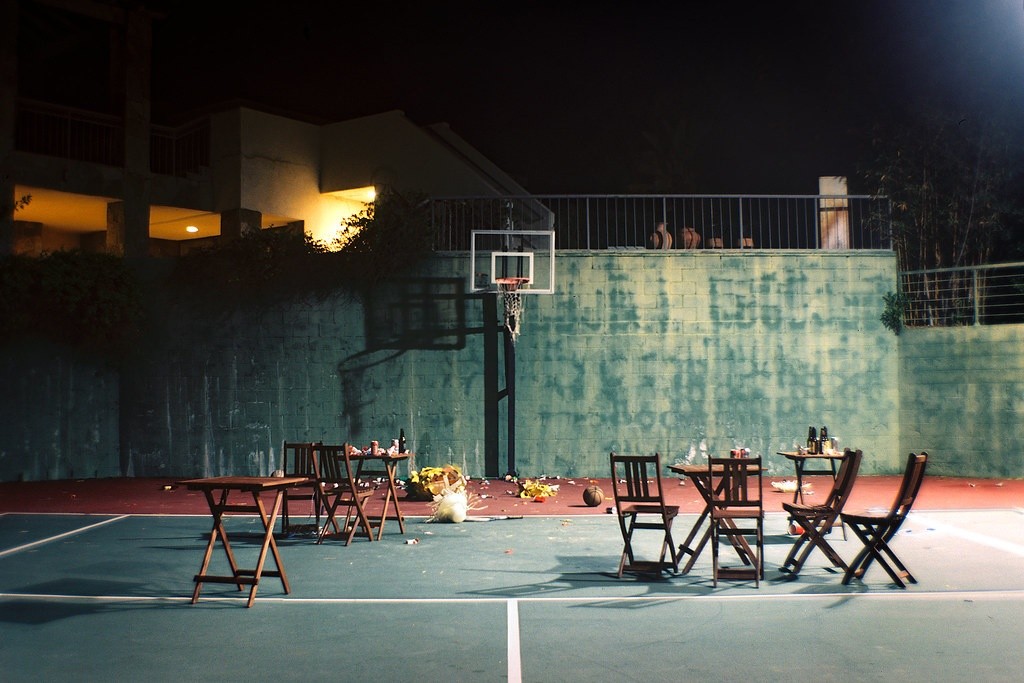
789;524;803;535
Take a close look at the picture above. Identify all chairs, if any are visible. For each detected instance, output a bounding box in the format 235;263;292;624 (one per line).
708;454;764;589
840;451;929;584
779;448;864;579
279;440;324;533
310;441;374;545
609;452;679;580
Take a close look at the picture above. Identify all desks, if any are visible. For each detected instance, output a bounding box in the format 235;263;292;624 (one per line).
332;454;414;541
666;463;763;572
776;451;847;541
172;477;306;605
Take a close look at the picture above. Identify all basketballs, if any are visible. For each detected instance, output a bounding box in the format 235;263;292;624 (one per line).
582;485;604;506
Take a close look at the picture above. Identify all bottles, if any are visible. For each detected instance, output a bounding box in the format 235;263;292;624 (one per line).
807;427;813;454
820;428;826;454
810;427;818;454
824;426;830;454
399;428;406;453
650;222;672;249
